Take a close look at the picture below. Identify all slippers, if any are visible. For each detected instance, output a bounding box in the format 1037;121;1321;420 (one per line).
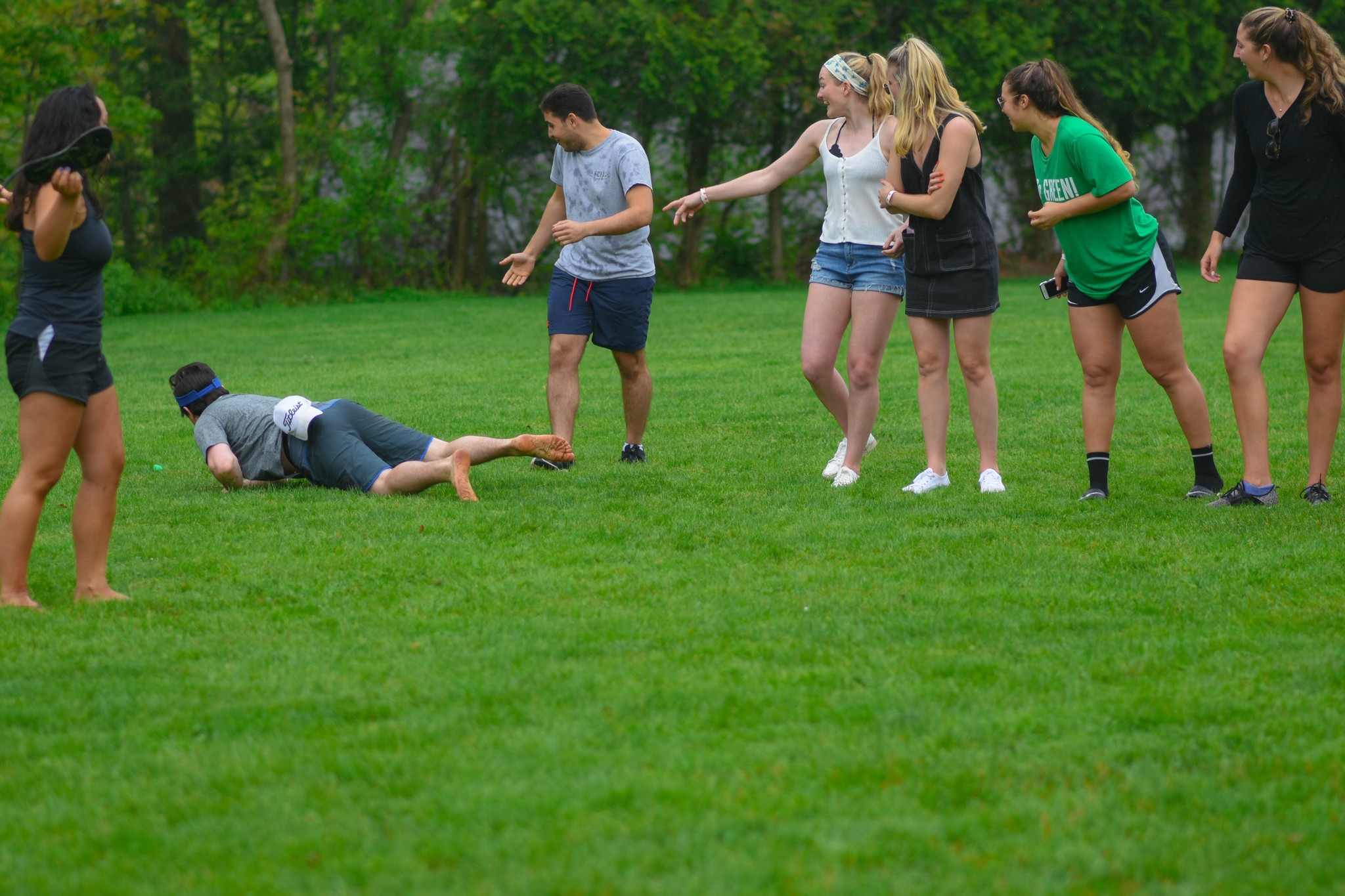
1182;485;1226;500
1077;488;1107;501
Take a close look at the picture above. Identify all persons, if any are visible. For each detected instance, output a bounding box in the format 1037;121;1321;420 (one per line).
878;36;1006;493
997;58;1224;502
662;51;915;488
500;82;654;470
1199;7;1345;508
0;88;129;608
170;363;566;499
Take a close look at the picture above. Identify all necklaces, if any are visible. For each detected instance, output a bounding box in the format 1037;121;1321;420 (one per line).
1275;102;1282;112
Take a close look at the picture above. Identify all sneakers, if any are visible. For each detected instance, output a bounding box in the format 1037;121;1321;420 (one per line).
831;466;860;487
978;468;1005;493
618;443;646;463
1300;473;1333;506
1205;478;1279;507
902;467;950;494
822;433;878;480
530;458;574;471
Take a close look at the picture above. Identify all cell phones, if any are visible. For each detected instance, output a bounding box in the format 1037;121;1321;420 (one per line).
1039;275;1069;299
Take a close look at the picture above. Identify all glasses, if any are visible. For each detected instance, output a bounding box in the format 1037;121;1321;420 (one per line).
997;94;1022;108
1265;117;1281;159
883;82;899;95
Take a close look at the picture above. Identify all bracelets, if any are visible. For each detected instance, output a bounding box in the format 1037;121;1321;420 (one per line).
700;188;709;204
886;189;898;207
1061;253;1065;259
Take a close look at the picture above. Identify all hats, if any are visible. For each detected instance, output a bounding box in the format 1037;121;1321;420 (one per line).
273;396;323;441
172;375;222;417
